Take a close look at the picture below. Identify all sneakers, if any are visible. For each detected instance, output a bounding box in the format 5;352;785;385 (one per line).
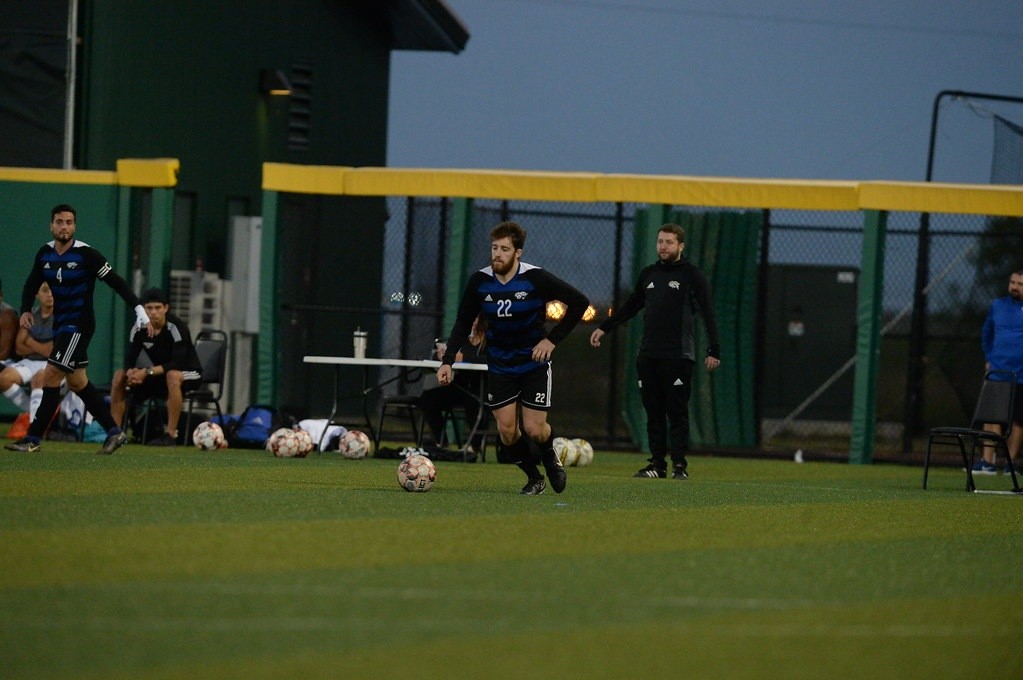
634;464;667;478
672;468;688;479
147;429;179;447
4;437;42;453
521;474;546;496
963;459;997;475
541;447;566;494
1003;462;1017;475
96;431;127;455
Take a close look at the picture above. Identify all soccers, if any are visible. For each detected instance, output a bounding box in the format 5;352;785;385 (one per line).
339;430;370;459
553;437;593;468
268;428;312;458
397;455;436;493
193;421;224;451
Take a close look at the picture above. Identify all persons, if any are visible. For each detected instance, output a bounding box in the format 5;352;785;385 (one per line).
419;312;489;462
963;270;1023;476
0;280;61;424
3;204;154;455
110;287;204;446
436;222;590;495
590;224;721;479
0;279;18;360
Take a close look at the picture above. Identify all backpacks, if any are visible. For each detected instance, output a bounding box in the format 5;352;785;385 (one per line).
229;405;277;448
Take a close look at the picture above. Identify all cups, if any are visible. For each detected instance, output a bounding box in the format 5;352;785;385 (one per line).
353;331;368;360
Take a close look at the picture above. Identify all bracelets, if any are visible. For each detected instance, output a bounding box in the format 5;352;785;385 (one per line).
146;367;153;375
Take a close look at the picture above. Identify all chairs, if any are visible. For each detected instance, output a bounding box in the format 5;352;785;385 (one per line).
372;337;501;462
921;368;1020;491
79;327;227;446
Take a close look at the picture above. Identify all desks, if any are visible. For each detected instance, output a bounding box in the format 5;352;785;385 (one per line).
303;355;488;460
